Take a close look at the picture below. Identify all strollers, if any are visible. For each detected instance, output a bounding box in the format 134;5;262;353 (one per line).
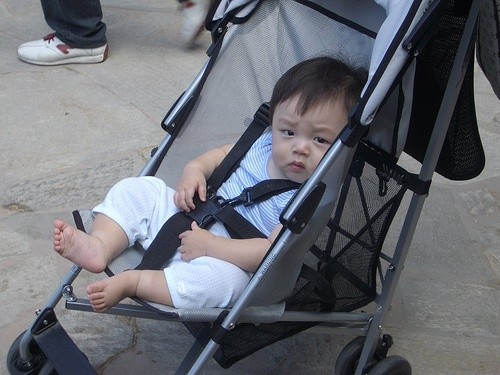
5;0;499;375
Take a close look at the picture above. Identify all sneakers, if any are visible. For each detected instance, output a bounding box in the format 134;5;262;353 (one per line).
179;0;215;48
16;31;107;66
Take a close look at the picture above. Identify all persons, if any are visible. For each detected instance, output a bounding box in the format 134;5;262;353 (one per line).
18;1;212;66
52;55;369;313
